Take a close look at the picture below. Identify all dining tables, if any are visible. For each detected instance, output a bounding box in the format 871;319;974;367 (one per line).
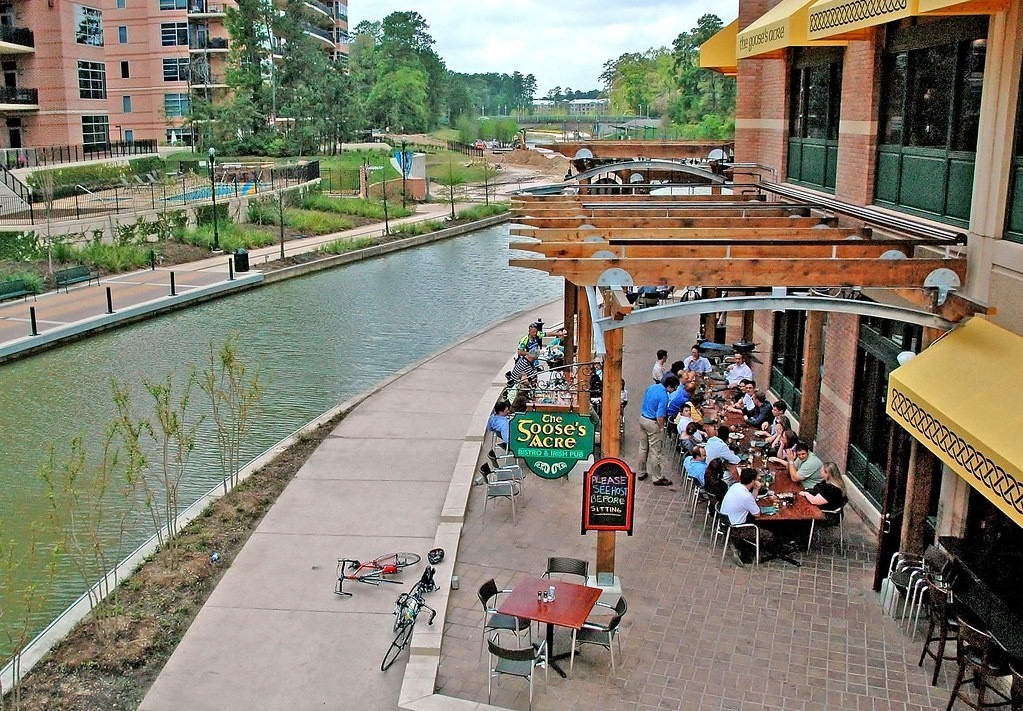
499;336;626;449
659;362;847;566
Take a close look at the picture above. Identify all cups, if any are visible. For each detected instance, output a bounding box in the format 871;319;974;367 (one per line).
772;497;780;510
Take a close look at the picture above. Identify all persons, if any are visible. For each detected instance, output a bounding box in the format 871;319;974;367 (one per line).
635;286;659;308
637;345;846;566
487;323;628;452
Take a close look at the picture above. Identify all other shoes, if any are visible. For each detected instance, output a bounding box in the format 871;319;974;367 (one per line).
638;473;648;480
653;477;673;487
733;550;746;568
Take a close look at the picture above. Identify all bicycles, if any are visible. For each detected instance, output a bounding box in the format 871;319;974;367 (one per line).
381;594;437;671
395;565;440;606
335;552;422;597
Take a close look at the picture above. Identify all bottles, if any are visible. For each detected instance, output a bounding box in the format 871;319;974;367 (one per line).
697;367;797;504
543;591;549;603
537;591;542;604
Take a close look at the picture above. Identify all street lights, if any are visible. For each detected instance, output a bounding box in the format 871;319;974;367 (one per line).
209;147;223;254
577;120;580;143
116;126;122;153
400;137;407;209
563;120;566;143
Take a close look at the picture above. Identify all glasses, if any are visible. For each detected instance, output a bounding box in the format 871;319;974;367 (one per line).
775;420;782;425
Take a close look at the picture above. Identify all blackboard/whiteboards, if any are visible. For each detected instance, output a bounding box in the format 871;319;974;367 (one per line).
581;457;636;537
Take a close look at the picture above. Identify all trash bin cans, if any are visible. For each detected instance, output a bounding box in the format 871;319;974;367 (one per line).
234;248;249;272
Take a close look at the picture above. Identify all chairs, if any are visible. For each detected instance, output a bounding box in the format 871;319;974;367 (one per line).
569;593;628;681
476;577;532;664
623;286;701;309
479;461;522;526
491;429;524;468
486;449;528;508
882;546;1023;711
487;631;549;710
539;556;589;589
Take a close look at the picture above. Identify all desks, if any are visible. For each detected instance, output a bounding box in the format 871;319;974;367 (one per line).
497;575;604;680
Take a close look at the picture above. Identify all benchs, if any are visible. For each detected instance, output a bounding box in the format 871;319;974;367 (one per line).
54;265;101;295
1;280;37;306
